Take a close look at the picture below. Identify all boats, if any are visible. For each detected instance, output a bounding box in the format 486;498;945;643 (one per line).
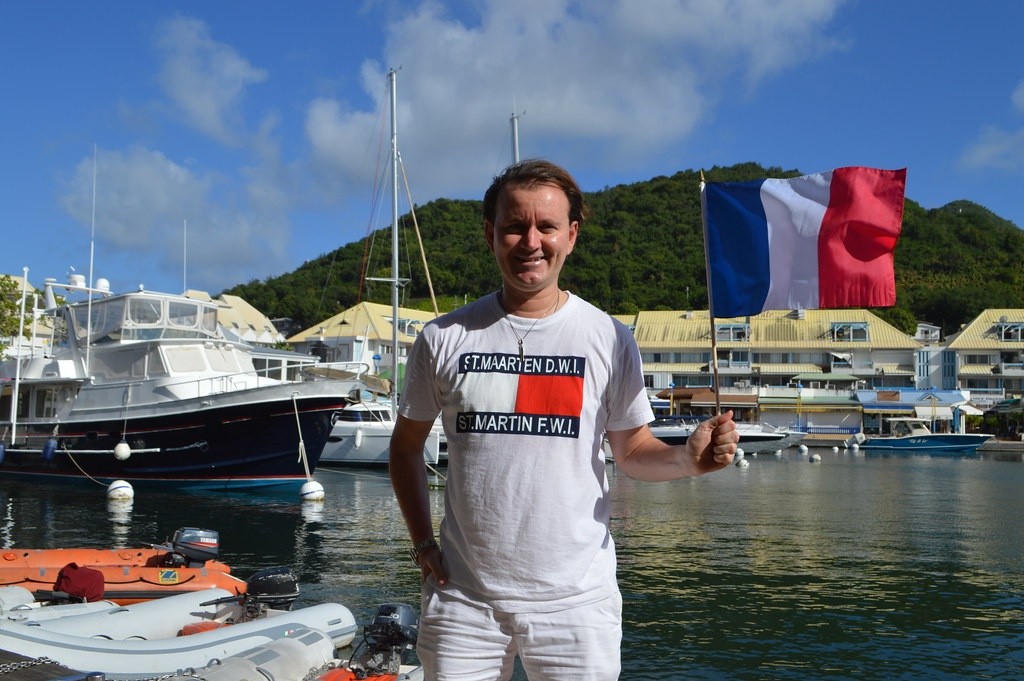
0;64;449;486
844;417;995;453
648;415;808;456
0;526;424;681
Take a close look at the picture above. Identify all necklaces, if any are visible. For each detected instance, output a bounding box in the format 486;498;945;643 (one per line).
499;287;560;362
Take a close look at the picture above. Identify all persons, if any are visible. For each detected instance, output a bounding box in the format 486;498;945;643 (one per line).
388;159;741;681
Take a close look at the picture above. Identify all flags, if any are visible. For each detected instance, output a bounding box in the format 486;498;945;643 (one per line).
699;166;907;318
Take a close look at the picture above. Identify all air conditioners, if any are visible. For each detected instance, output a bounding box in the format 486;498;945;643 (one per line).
734;379;750;388
